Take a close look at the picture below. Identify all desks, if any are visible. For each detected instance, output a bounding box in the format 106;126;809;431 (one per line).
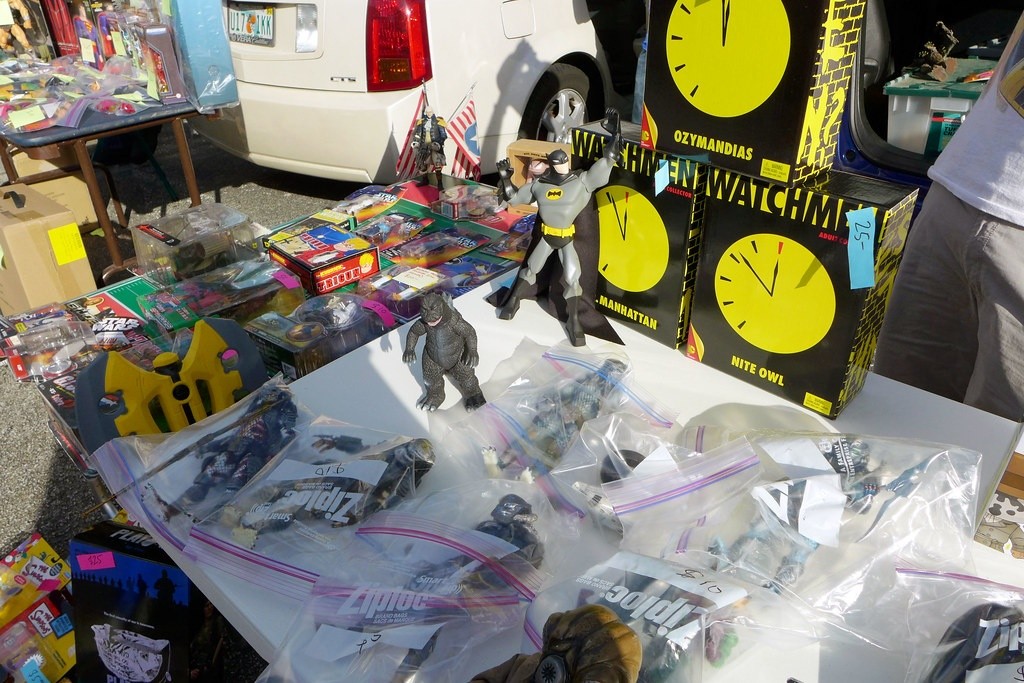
156;263;1023;682
0;95;222;289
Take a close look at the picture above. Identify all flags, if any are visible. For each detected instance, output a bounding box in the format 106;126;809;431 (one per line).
444;93;483;182
395;88;435;183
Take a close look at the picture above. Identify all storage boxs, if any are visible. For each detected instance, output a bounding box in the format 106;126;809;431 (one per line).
135;0;237;107
973;419;1023;558
0;142;96;316
244;290;395;383
570;118;711;350
0;532;77;683
269;222;380;294
504;137;571;215
69;514;226;682
688;169;920;420
260;206;358;250
881;58;998;153
638;0;868;190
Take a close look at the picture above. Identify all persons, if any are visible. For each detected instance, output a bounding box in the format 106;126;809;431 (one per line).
870;11;1024;425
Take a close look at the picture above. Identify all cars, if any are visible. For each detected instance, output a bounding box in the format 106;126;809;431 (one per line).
171;0;636;185
